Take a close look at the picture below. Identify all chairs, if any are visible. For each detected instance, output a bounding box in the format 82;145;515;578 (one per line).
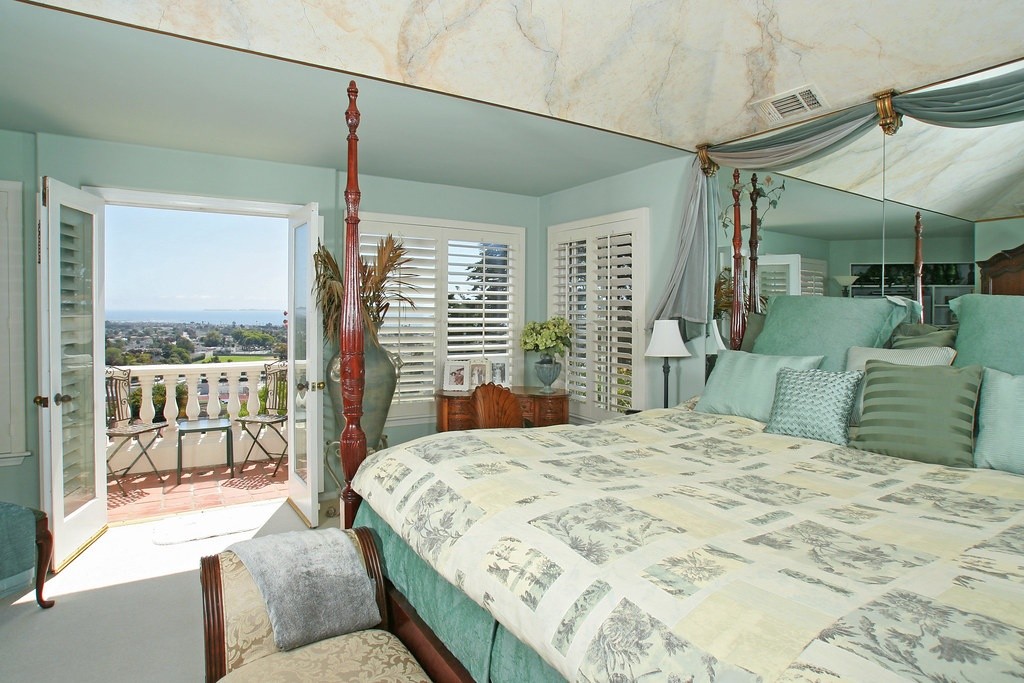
105;364;169;498
465;382;525;429
234;357;288;478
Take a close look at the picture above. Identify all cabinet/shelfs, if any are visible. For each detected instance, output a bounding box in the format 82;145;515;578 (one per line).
849;262;976;328
435;385;572;435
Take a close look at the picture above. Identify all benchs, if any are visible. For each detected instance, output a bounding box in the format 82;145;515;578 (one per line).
196;524;434;683
0;502;54;610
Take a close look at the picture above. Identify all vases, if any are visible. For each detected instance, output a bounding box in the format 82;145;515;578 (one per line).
309;319;421;451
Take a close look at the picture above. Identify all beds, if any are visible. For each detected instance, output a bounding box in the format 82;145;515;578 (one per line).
341;80;1024;683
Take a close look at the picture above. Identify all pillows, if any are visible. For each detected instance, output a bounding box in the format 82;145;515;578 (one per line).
751;293;907;372
846;345;957;374
691;348;826;423
972;366;1024;475
897;296;923;324
948;292;1024;376
890;327;957;349
739;311;766;353
763;366;865;446
847;359;984;469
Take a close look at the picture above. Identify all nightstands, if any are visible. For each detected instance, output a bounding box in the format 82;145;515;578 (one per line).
625;408;642;415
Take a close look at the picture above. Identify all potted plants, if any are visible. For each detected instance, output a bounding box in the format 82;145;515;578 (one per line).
518;315;573;394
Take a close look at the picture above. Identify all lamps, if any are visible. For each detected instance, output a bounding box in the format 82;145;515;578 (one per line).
831;275;860;297
643;320;691;408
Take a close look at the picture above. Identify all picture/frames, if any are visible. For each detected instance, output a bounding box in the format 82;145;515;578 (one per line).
470;356;493;390
491;359;509;387
443;361;471;391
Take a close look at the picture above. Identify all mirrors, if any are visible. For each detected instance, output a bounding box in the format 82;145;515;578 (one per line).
703;57;1024;385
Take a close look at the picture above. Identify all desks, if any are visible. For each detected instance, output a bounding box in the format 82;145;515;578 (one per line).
177;418;235;485
